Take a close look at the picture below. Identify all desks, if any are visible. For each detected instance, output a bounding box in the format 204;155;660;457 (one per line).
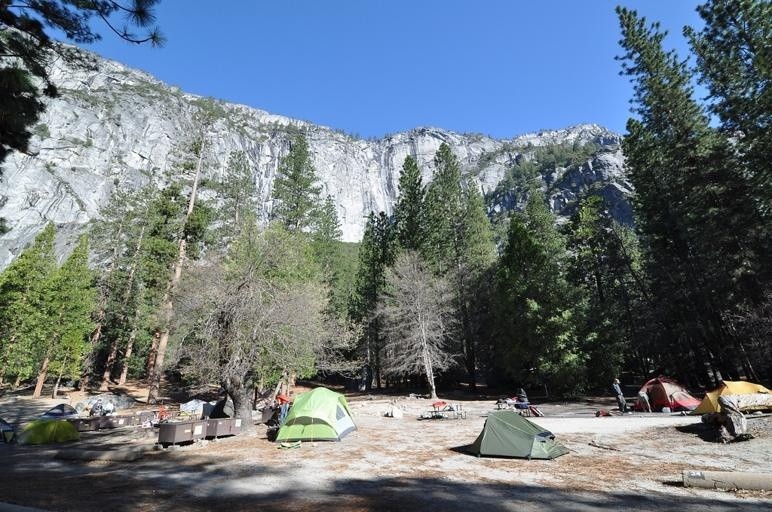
67;408;238;446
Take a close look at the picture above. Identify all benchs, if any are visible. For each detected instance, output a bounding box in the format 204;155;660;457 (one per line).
425;401;532;419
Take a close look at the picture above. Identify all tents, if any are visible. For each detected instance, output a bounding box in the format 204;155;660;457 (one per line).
16;418;81;444
275;386;358;442
44;404;78;418
467;411;569;460
633;375;702;412
689;379;772;415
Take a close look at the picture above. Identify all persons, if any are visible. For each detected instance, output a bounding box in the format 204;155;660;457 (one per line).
105;400;115;414
611;379;629;413
90;399;104;416
638;391;652;413
